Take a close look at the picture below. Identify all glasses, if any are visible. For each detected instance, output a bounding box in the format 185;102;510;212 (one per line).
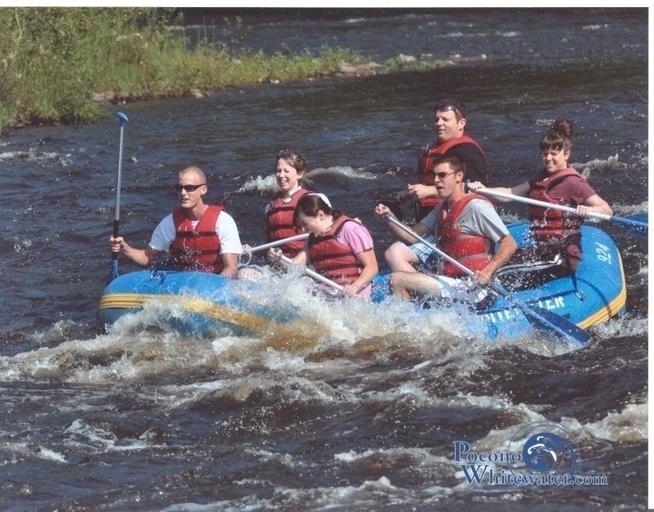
429;168;464;180
174;182;207;192
432;100;464;118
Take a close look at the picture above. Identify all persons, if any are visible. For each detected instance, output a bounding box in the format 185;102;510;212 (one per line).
238;147;314;284
389;97;489;224
266;192;380;302
110;164;244;279
374;153;518;310
466;116;614;293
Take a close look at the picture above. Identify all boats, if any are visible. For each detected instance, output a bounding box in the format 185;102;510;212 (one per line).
99;223;631;354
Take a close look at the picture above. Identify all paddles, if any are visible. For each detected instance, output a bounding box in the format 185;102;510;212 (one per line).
379;210;590;347
466;183;648;237
102;111;130;283
